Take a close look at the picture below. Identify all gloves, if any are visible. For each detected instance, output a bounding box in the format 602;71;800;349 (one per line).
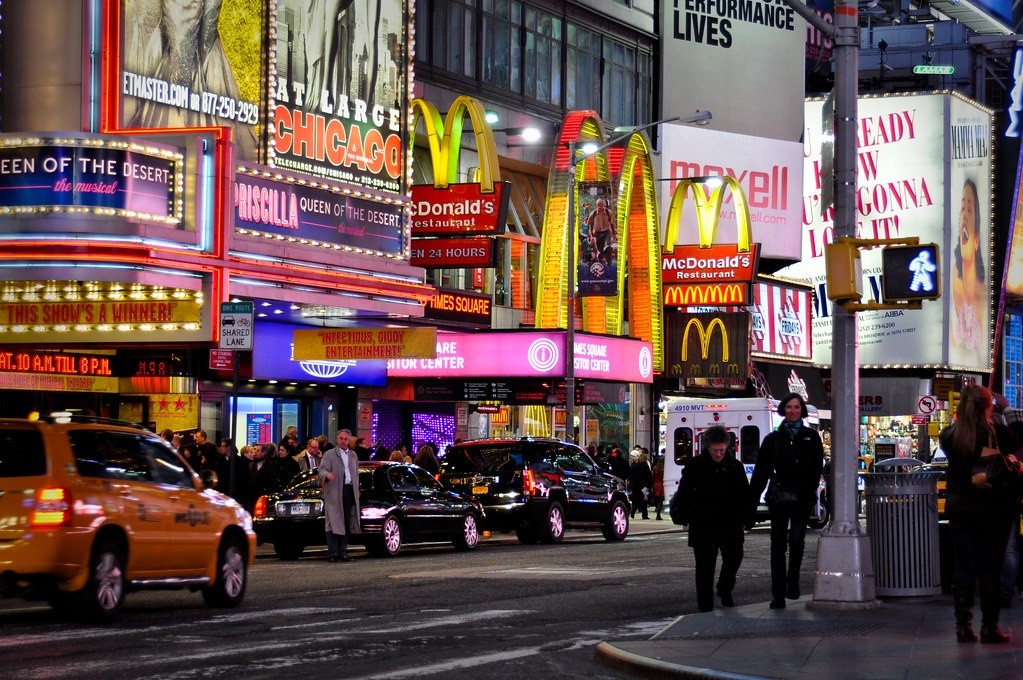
988;391;1009;415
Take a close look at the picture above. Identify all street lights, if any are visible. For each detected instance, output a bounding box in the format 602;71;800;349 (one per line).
564;108;714;445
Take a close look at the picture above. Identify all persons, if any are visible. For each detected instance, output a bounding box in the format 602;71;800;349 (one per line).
669;426;749;613
318;429;361;562
953;180;988;355
937;387;1023;645
159;427;442;546
585;443;666;519
749;393;824;609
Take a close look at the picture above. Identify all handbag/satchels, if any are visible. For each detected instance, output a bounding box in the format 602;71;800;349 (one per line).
670;457;695;527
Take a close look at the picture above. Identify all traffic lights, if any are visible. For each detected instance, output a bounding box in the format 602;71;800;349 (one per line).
880;242;943;303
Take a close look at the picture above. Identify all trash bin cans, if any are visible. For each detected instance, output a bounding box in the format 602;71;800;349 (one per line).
858;457;945;597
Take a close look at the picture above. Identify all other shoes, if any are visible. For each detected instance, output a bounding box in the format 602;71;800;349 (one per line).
656;517;663;520
786;570;801;600
769;596;784;609
341;553;355;562
329;555;342;564
631;511;635;518
716;587;735;607
643;516;649;519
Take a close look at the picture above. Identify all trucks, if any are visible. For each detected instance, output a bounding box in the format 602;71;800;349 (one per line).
663;397;829;530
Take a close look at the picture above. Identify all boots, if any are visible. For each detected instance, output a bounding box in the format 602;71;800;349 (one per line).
956;612;977;642
980;608;1010;644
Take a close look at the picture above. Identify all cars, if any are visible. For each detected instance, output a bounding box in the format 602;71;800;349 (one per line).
908;457;949;520
253;460;486;563
0;413;258;626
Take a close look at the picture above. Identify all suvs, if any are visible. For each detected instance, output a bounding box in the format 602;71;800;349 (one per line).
435;435;634;546
